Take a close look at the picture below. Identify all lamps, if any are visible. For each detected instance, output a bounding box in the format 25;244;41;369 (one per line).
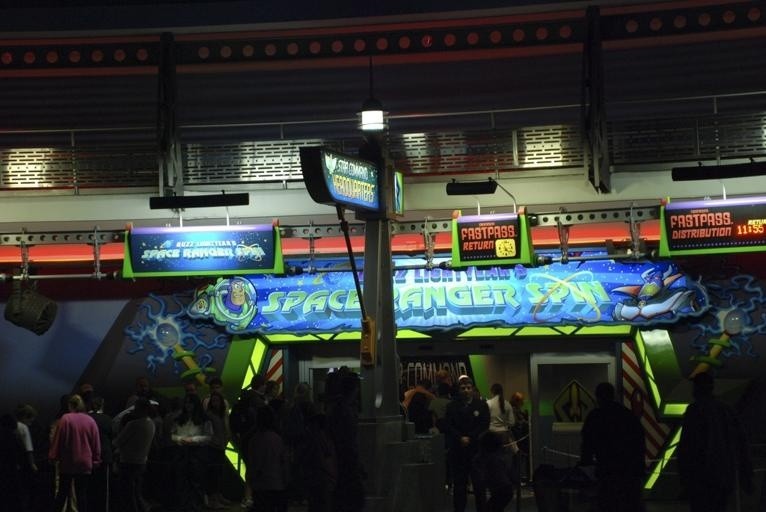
671;158;765;199
446;178;517;214
150;191;251;227
356;31;389;133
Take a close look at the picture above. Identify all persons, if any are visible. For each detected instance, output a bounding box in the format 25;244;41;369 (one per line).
241;376;356;511
579;380;643;511
1;385;112;511
673;370;754;512
112;377;232;511
399;374;529;512
